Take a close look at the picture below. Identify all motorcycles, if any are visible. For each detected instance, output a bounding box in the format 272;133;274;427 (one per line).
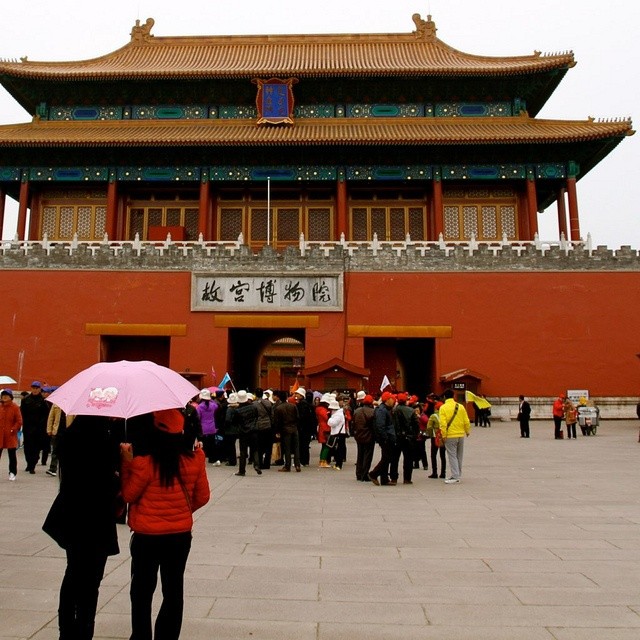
580;412;599;434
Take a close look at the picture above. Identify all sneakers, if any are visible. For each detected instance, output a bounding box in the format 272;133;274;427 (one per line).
444;478;460;484
46;470;57;476
439;473;445;478
8;472;15;480
319;461;331;467
212;460;221;467
428;472;438;478
403;479;414;484
387;480;397;485
368;473;379;485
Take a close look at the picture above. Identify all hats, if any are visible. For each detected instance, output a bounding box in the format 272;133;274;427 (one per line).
409;395;418;403
434;401;443;408
398;393;407;401
153;409;185;433
227;393;237;404
1;389;13;399
41;387;50;392
328;401;340;410
236;390;248;403
559;393;565;399
50;387;57;393
319;392;336;403
199;389;211;400
30;381;40;386
382;392;396;401
356;390;366;400
363;395;373;404
294;388;306;399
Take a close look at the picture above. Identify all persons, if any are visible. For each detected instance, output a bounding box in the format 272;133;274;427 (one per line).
512;395;533;439
20;380;49;474
20;390;29;401
386;392;421;486
473;393;482;428
326;399;347;470
350;394;377;482
119;410;211;640
438;388;471;484
36;386;54;467
425;401;447;478
635;399;640;444
187;385;445;472
249;386;276;470
230;390;263;477
550;393;566;440
482;393;493;428
367;390;398;486
41;414;126;640
0;388;26;479
273;390;302;472
45;403;78;477
562;395;578;441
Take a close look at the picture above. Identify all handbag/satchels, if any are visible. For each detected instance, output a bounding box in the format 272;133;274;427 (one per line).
358;406;374;448
517;412;522;421
435;404;459;447
326;424;343;451
271;437;281;463
420;414;430;437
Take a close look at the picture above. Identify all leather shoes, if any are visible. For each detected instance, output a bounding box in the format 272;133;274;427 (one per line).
235;472;245;475
296;466;301;471
255;466;262;474
278;467;290;471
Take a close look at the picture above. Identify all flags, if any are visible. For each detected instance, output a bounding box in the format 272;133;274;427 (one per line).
216;371;238;393
290;379;303;394
465;389;491;412
378;373;394;392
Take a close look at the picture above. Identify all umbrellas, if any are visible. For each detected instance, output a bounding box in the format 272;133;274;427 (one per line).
0;371;18;391
46;358;202;453
